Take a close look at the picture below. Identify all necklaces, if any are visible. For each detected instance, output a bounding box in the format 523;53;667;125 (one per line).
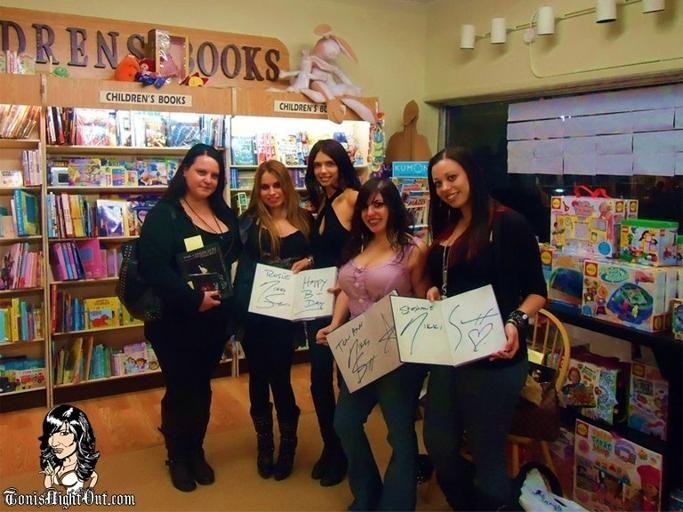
179;195;224;243
440;224;473;298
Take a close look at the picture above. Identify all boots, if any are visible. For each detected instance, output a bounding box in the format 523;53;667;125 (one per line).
156;400;196;492
196;392;215;485
311;385;349;486
249;402;276;479
275;406;300;481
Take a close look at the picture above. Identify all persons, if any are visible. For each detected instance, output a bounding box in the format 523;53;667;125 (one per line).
315;179;424;511
421;144;550;510
232;161;320;480
302;140;366;486
141;141;242;491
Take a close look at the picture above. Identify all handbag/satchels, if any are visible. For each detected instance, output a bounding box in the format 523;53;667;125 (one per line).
513;361;560;441
115;239;164;326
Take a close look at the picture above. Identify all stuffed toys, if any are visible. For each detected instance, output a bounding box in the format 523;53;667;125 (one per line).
117;26;213;90
283;27;373;129
506;184;683;511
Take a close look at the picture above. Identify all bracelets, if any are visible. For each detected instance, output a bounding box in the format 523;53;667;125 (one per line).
503;307;532;334
304;256;315;266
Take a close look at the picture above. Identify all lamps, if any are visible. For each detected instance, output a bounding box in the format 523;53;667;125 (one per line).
458;0;668;50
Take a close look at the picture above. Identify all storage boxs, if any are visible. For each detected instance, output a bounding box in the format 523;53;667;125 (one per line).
538;186;682;338
545;342;669;512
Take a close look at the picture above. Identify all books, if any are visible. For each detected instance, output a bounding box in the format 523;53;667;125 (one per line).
0;106;430;395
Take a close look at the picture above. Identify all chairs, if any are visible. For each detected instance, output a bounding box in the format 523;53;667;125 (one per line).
507;307;572;497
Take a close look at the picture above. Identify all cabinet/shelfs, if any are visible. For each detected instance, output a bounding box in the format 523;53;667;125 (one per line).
0;71;50;406
502;288;682;512
45;78;235;405
233;88;373;217
396;175;429;239
